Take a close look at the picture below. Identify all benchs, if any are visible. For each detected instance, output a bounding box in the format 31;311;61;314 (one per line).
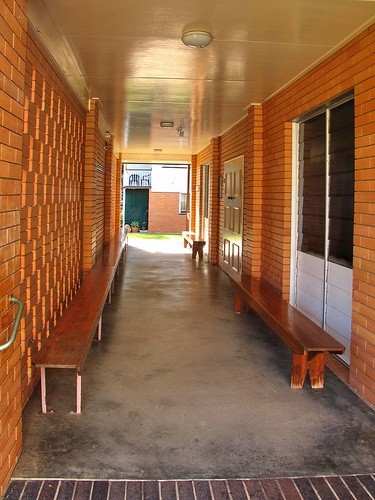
182;230;206;260
33;227;130;413
230;273;343;389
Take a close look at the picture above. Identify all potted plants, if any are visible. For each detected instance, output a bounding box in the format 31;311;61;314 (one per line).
130;221;141;233
